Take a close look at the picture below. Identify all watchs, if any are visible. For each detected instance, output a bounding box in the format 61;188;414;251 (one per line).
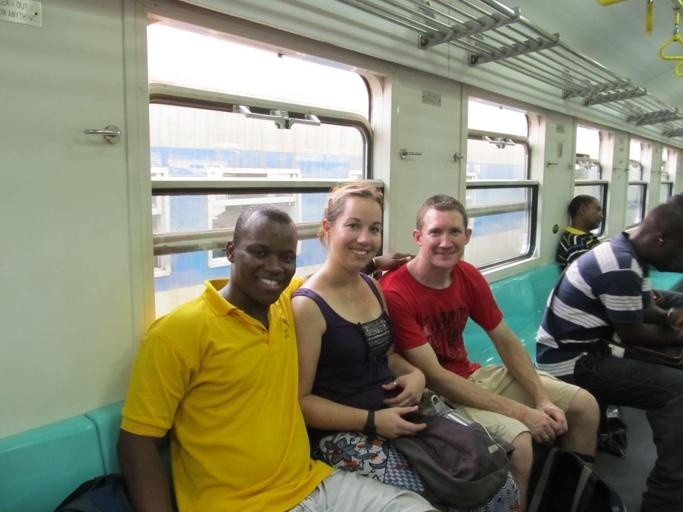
362;407;377;436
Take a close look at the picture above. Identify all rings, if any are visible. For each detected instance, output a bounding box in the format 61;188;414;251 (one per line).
392;380;397;387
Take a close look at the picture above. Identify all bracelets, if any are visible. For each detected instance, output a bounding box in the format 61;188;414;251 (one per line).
371;256;378;268
665;307;681;331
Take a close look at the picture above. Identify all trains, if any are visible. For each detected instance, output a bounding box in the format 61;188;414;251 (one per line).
149;115;674;322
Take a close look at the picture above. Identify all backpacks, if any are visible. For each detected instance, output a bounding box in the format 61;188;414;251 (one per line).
384;382;512;509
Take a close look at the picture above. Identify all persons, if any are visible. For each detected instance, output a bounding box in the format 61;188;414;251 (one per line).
374;194;602;511
117;203;441;511
555;195;682;309
533;199;681;510
288;178;524;511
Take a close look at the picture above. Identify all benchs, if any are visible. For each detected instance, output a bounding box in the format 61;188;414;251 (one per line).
1;394;175;507
450;248;683;418
